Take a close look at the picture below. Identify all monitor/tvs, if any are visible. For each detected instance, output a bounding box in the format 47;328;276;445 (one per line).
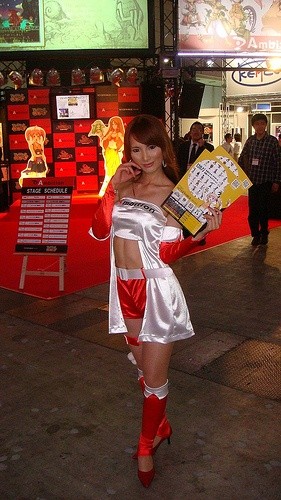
56;95;90;120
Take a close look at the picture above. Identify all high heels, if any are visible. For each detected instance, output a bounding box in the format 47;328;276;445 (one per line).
130;426;173;458
137;453;155;488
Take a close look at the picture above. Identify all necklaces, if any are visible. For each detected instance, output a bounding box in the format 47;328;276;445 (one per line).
132;182;136;197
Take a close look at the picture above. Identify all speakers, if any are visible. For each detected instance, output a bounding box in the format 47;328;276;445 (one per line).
139;80;165;120
178;79;205;119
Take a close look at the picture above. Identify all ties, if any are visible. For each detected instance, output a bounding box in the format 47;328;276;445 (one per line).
189;143;197;164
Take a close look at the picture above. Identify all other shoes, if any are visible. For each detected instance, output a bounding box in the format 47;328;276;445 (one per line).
251;236;260;246
259;232;269;245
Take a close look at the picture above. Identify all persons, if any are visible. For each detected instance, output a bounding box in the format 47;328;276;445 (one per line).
238;114;281;246
223;133;234;158
233;133;242;161
87;115;223;489
177;121;214;245
